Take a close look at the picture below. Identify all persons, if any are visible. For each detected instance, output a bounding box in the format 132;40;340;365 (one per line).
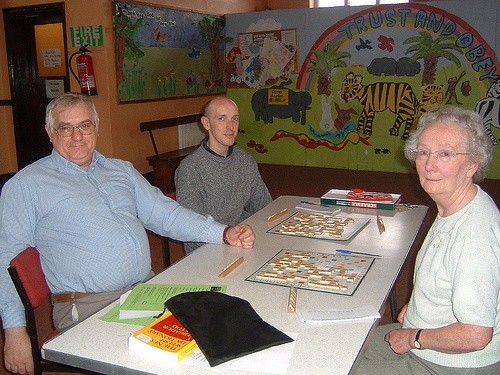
349;103;499;374
0;92;256;375
174;96;272;254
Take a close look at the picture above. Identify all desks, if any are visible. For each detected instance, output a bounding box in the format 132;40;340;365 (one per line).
40;196;429;375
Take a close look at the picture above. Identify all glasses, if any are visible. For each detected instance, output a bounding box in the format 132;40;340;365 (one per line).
53;122;96;137
410;149;471;162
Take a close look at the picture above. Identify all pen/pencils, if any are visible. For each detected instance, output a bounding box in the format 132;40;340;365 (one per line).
300;201;329;207
336;249;382;256
377;215;386;232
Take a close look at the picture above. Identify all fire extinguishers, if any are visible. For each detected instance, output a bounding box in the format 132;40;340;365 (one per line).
69;43;99;97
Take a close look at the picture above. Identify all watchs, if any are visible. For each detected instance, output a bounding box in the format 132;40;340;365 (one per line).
223;225;233;245
414;329;425;350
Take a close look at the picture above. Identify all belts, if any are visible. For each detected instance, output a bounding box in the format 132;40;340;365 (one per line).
52;292;97;303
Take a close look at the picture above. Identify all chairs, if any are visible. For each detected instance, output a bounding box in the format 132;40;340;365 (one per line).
161;192;188;268
6;246;102;375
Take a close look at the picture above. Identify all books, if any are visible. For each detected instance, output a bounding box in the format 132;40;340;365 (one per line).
129;308;198;363
118;283;230;319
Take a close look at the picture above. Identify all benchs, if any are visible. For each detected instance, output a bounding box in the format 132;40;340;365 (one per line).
138;112;206;194
